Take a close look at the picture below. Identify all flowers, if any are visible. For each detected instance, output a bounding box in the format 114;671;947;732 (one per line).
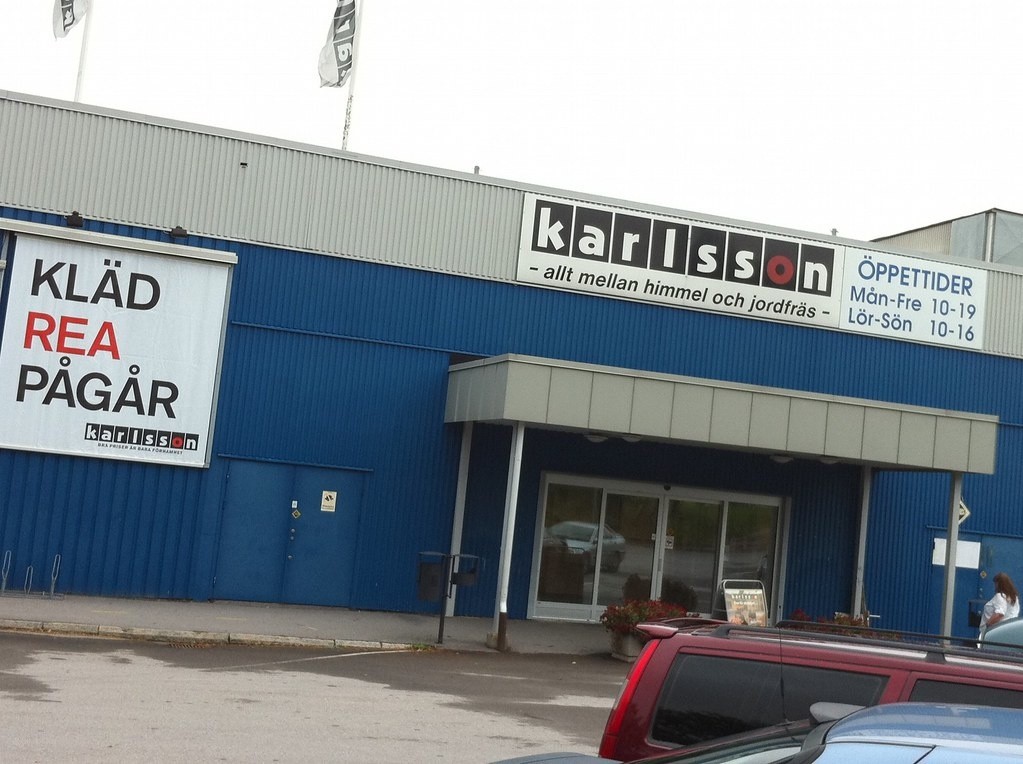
783;609;902;641
598;598;703;638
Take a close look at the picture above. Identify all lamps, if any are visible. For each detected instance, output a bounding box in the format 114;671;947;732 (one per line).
169;226;187;240
67;211;83;227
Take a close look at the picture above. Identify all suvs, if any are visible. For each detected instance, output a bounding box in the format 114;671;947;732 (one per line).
597;615;1022;760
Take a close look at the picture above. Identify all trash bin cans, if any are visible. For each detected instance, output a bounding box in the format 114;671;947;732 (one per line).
413;549;482;599
966;599;990;628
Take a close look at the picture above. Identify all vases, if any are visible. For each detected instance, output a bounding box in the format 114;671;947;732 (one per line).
611;629;647;662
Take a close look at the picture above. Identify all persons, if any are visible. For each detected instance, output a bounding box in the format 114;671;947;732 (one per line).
979;573;1020;649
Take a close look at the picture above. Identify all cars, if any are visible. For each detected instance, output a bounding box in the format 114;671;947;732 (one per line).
483;702;1022;764
548;521;628;574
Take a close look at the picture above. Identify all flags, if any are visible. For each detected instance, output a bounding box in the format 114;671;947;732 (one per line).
315;1;359;89
52;0;87;38
